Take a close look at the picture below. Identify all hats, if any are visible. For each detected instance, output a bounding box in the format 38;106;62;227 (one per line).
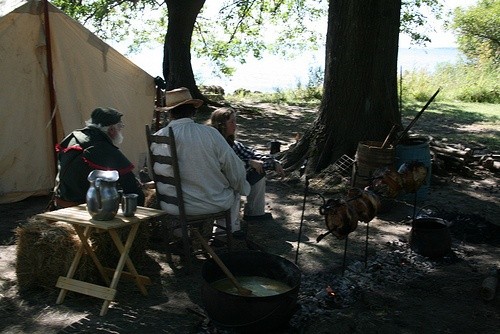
155;87;204;112
91;107;123;127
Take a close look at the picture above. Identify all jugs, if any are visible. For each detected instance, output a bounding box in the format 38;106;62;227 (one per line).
86;170;120;221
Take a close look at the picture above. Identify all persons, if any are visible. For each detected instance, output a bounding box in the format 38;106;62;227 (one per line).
209;107;283;220
52;108;146;211
150;87;252;240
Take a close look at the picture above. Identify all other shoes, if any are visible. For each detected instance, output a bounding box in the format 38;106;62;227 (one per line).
233;230;245;240
243;213;273;222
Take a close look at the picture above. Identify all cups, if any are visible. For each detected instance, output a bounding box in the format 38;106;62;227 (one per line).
122;194;138;217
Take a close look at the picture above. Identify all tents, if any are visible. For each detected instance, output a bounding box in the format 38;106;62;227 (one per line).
0;0;156;204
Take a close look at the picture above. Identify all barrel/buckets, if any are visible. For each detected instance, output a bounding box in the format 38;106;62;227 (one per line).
352;140;395;190
393;134;434;201
201;250;301;331
408;204;451;257
